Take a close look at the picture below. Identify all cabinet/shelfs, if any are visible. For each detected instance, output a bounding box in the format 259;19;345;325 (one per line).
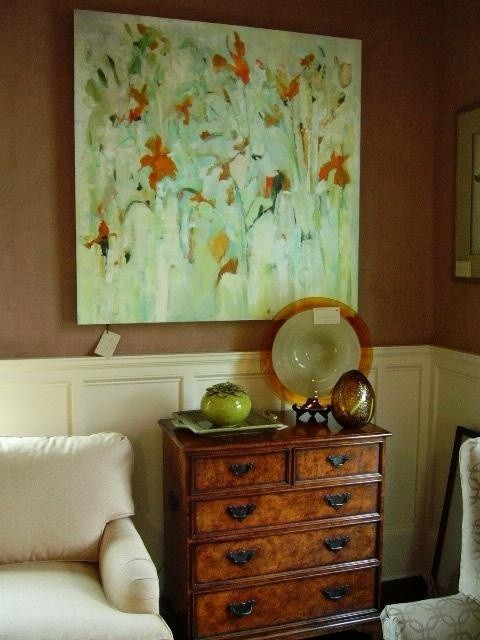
154;404;399;638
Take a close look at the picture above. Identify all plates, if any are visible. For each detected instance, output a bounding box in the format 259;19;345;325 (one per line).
170;407;287;436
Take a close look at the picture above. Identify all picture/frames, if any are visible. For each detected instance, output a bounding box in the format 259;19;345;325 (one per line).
453;102;480;283
426;423;478;599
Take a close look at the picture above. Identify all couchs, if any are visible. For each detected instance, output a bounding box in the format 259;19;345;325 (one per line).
0;433;175;640
376;434;479;640
